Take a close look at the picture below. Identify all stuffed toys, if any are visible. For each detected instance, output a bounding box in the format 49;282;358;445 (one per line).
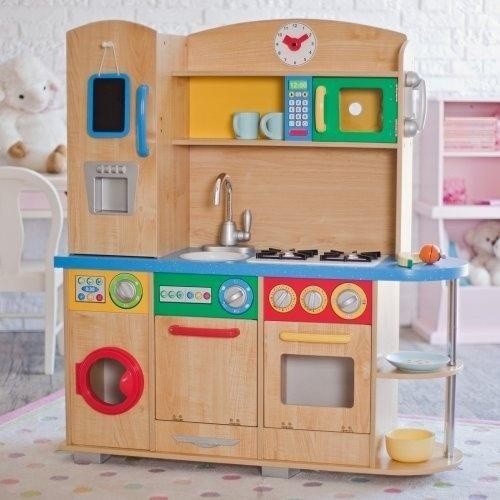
466;222;500;284
0;57;68;172
